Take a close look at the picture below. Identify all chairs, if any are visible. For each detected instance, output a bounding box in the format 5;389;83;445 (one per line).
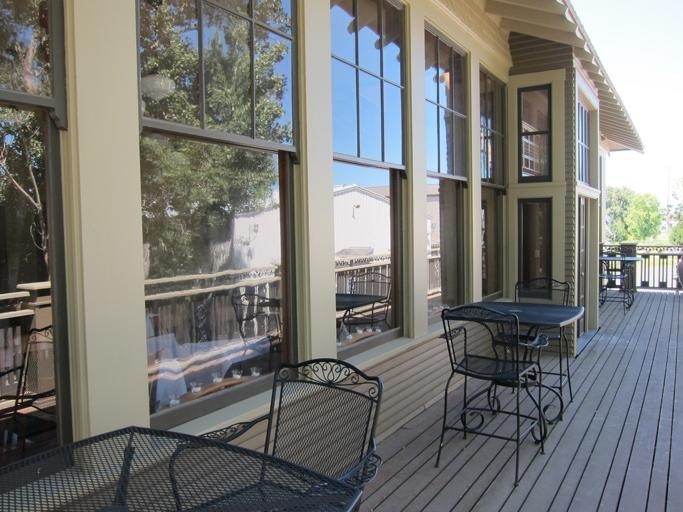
231;293;286;357
0;325;58;449
434;277;574;486
168;357;384;512
599;252;635;315
342;272;393;334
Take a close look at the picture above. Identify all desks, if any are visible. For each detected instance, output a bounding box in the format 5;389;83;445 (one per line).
0;425;362;512
598;255;640;303
335;293;387;335
441;301;585;444
